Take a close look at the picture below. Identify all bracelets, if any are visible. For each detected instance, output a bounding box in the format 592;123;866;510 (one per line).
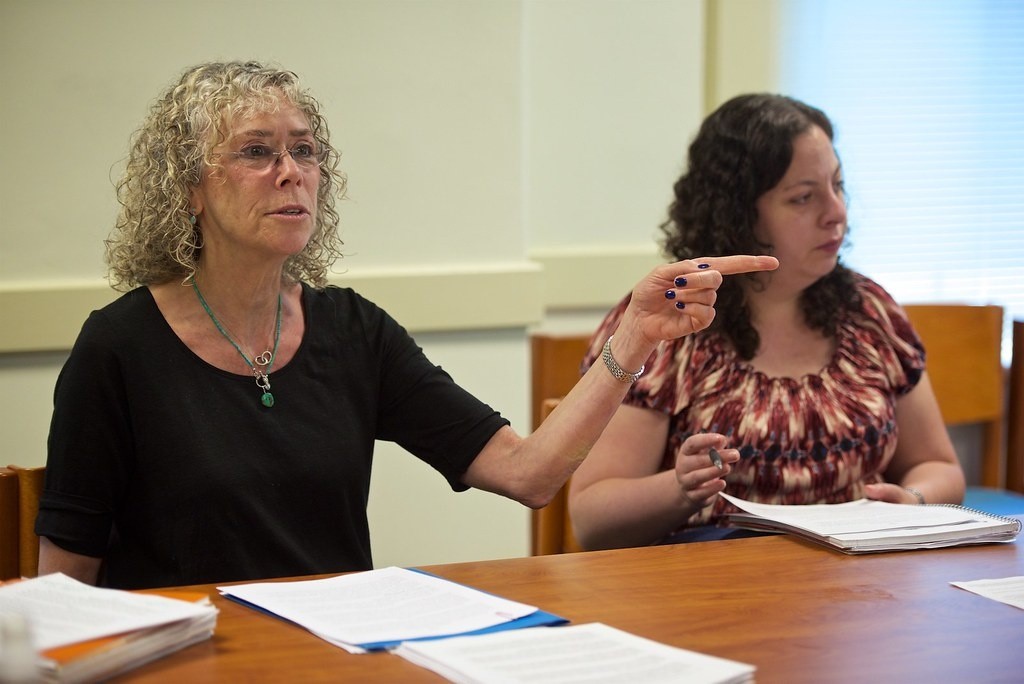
602;335;645;382
904;486;925;504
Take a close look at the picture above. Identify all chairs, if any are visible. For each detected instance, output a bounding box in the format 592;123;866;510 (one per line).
0;464;45;582
899;304;1005;492
529;332;594;556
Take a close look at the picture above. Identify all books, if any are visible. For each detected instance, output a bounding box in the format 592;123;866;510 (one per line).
718;489;1021;556
0;572;221;683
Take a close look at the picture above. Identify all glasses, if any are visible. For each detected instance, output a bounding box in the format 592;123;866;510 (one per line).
208;141;330;170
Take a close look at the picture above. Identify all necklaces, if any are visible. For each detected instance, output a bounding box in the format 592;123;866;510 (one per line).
188;275;282;407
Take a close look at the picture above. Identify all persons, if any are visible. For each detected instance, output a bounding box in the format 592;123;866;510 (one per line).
34;58;780;592
566;91;966;552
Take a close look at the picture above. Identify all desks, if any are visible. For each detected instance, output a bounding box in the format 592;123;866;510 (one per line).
102;514;1024;684
